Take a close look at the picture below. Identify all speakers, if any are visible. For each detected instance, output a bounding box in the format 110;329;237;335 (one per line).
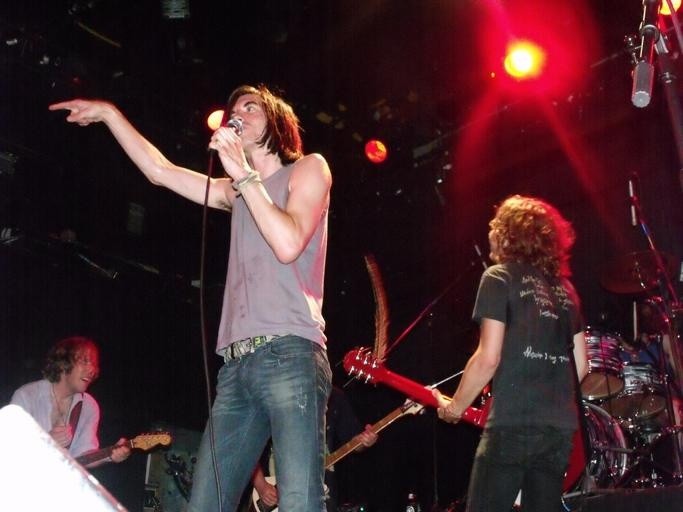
141;422;204;512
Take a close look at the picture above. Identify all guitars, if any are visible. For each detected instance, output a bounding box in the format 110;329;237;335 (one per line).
78;426;175;470
251;391;425;512
342;345;592;493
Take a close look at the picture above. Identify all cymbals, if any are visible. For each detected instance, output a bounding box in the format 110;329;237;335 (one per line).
600;250;680;296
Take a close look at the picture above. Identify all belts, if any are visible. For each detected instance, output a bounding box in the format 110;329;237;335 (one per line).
223;332;291;364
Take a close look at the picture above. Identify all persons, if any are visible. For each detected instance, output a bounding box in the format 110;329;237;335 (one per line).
435;188;590;511
247;416;378;512
46;80;335;511
10;332;131;476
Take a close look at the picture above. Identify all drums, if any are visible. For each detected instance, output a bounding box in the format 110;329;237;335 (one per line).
579;329;625;401
581;402;630;493
634;412;672;439
616;415;637;435
598;361;668;422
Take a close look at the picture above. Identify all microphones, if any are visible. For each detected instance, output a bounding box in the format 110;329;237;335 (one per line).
473;242;489;271
209;118;242;156
626;171;640;225
629;0;662;108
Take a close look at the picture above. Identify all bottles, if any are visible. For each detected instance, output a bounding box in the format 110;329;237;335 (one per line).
404;486;420;512
